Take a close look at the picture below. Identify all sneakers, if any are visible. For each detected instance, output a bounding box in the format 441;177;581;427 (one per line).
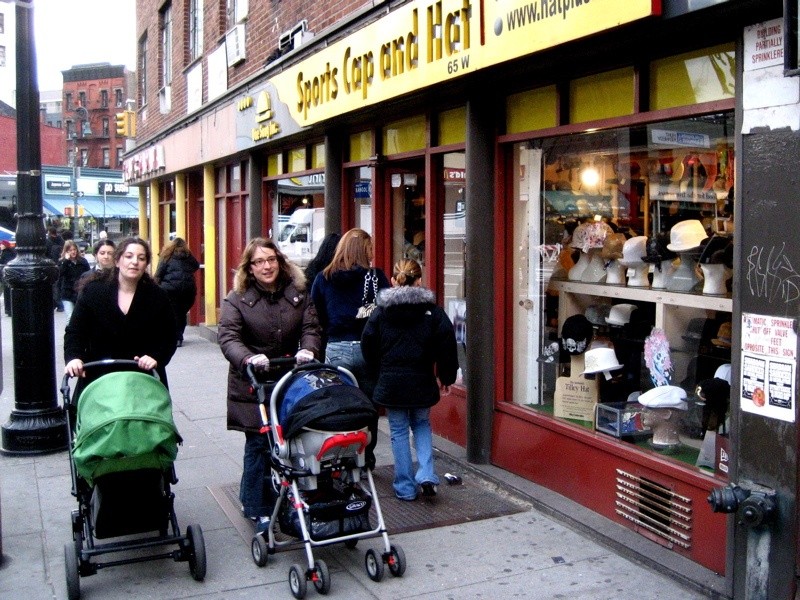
240;505;279;534
421;482;438;496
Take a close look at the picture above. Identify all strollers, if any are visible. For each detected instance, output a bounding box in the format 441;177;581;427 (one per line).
245;356;407;600
59;358;209;600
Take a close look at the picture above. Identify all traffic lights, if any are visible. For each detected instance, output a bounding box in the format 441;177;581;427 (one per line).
65;207;74;215
115;111;128;138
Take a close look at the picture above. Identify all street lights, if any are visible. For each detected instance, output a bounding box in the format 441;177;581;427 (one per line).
66;106;93;244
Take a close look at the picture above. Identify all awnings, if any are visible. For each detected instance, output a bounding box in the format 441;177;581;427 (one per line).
41;195;176;220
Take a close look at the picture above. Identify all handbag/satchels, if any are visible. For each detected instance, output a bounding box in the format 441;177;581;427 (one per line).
355;303;377;328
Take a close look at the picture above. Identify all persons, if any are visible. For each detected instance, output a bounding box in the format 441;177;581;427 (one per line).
218;238;320;536
153;233;199;348
0;227;73;317
64;238;179;438
53;239;91;327
99;231;108;239
79;239;117;298
304;234;342;291
563;220;735;446
311;228;392;472
358;256;463;501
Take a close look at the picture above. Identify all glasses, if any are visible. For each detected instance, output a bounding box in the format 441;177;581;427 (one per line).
249;257;277;266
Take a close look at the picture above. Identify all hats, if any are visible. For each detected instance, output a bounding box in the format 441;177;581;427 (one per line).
578;348;633;379
680;356;717;391
638;385;688;411
584;303;656;333
569;219;733;270
711;322;732;348
562;314;592;355
681;318;718;344
536;337;571;364
713;364;731;386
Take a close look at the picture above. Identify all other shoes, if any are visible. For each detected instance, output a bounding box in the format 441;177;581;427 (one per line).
361;469;372;478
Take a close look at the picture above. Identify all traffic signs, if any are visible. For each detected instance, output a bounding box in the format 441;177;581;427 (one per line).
70;191;84;197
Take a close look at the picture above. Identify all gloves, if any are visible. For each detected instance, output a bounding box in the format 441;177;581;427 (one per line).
245;354;270;374
294;349;314;366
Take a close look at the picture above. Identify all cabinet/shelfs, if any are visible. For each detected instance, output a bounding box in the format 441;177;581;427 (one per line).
546;277;733;452
